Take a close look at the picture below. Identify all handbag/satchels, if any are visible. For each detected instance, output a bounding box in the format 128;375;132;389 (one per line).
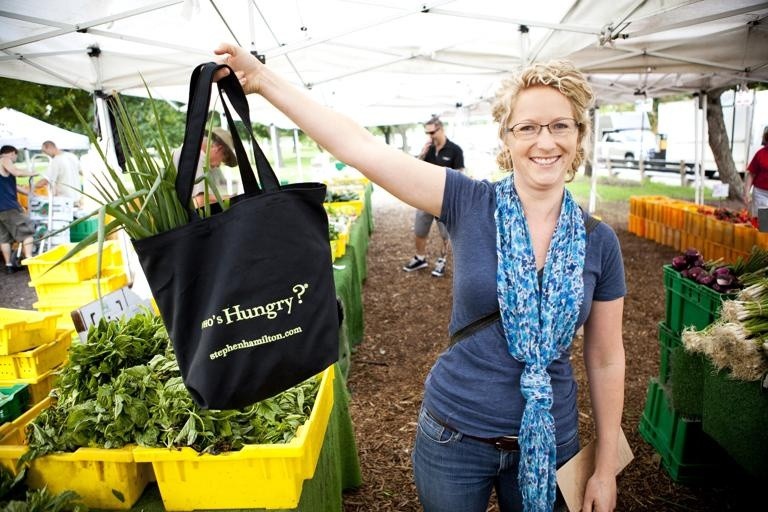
129;182;342;410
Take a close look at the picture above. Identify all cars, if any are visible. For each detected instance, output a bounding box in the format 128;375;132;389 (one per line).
594;126;660;168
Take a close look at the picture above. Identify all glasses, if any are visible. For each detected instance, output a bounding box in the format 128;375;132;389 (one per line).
424;126;440;135
506;116;578;142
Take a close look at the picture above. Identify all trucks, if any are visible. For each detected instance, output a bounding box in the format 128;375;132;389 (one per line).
646;90;768;177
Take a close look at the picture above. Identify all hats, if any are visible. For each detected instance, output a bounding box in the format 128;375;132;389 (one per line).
204;125;240;167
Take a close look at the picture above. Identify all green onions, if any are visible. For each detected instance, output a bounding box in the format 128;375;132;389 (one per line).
19;70;225;311
681;279;768;385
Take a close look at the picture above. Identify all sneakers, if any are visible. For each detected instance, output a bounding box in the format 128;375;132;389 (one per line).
431;257;447;277
403;254;429;273
5;267;25;274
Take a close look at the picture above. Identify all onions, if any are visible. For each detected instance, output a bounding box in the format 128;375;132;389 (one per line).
672;249;739;293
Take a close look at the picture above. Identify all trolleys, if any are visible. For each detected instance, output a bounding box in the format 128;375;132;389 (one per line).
11;147;62;270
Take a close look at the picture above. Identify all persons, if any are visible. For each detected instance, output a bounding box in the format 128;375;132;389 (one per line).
741;124;768;214
211;38;629;512
401;117;463;277
170;127;238;208
30;140;83;209
0;145;43;274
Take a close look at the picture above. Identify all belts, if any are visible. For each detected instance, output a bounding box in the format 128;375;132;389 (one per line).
425;411;521;452
